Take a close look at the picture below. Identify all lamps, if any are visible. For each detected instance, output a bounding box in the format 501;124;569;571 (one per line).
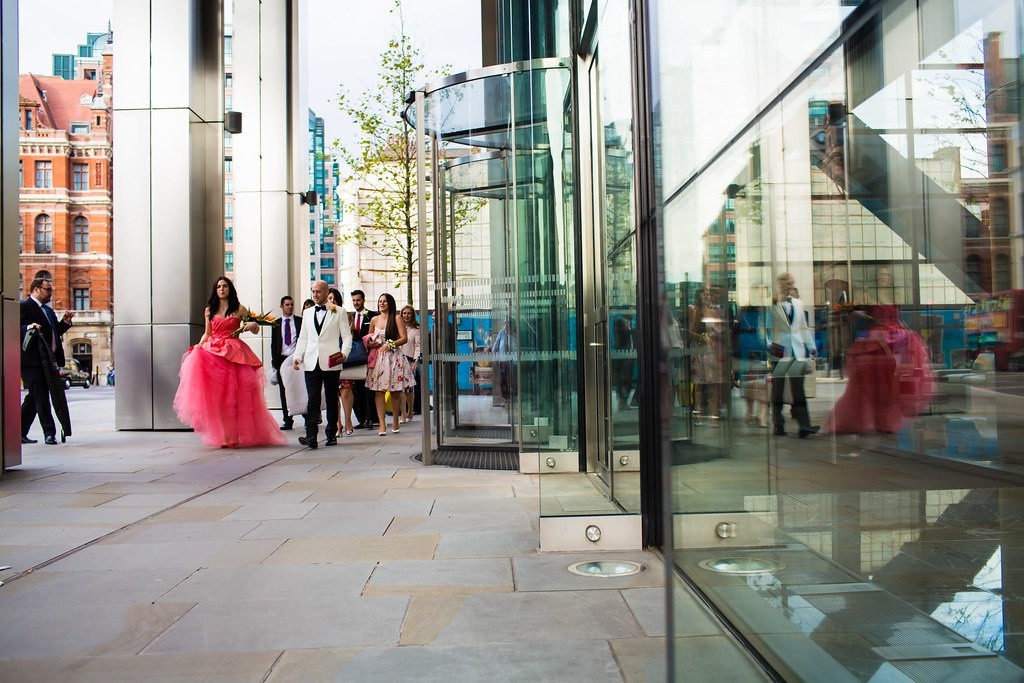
303;191;317;206
224;112;242;134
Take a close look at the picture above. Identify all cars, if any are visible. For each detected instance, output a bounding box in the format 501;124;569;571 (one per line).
59;358;90;389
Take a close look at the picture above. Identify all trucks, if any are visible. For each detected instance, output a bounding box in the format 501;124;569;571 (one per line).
962;290;1024;370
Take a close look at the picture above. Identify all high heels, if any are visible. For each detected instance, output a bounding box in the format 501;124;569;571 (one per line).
345;422;353;435
336;426;343;437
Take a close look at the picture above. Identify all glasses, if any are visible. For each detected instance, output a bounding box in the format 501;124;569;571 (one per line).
38;286;51;292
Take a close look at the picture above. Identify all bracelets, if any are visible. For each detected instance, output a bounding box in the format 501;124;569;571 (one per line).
386;339;396;353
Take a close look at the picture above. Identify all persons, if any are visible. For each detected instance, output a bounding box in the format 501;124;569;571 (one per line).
609;266;934;439
106;366;115;386
292;281;352;448
272;296;323;430
492;320;518;407
366;292;417;435
431;310;455;410
399;305;422;414
173;278;287;446
328;288;380;436
21;278;72;445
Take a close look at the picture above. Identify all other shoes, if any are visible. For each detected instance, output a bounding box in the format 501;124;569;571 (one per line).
363;419;373;428
379;429;386;436
221;443;228;448
279;424;292;430
392;428;399;433
500;399;507;407
399;417;412;423
695;421;702;426
353;421;364;429
710;423;718;427
230;442;239;446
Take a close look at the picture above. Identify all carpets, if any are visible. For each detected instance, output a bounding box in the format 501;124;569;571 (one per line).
433;450;520;470
671;441;728;466
447;428;511;439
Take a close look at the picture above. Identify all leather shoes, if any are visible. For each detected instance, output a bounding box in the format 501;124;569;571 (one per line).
799;424;820;438
22;436;38;444
299;436;318;449
45;435;57;445
774;424;787;436
326;434;337;446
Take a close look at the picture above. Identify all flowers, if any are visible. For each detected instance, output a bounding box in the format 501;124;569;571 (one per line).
772;295;779;305
231;307;280;338
828;300;880;324
329;305;337;315
363;313;370;320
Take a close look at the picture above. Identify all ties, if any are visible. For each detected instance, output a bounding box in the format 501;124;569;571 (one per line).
285;319;291;346
356;314;361;331
41;304;57;352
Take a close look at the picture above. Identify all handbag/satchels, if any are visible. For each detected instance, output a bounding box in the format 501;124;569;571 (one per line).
340;365;368;380
343;341;367;368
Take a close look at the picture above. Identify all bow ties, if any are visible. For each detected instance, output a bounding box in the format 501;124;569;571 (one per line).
781;297;792;303
315;305;326;311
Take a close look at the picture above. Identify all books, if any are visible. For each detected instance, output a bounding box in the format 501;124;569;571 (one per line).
328;352;343;368
